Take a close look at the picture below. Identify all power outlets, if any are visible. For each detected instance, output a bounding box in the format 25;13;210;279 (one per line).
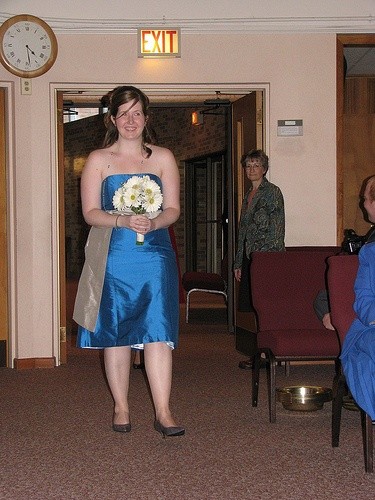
20;78;33;95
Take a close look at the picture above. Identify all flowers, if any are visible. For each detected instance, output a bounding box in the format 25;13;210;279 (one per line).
112;176;163;244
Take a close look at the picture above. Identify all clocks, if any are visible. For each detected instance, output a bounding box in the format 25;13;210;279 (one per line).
0;14;58;78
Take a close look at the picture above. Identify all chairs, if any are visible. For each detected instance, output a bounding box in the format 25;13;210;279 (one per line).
182;252;228;323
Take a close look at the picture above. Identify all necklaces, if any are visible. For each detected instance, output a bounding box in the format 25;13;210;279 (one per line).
102;141;144;215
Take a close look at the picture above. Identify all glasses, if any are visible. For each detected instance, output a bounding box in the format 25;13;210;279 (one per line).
245;163;263;169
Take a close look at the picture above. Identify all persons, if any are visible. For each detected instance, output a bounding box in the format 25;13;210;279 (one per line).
234;149;285;370
315;176;375;424
81;86;187;438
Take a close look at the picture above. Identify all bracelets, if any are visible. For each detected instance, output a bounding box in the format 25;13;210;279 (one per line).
116;214;121;228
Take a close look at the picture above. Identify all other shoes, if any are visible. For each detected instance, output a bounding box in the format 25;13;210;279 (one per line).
113;415;131;432
154;419;185;436
239;359;266;369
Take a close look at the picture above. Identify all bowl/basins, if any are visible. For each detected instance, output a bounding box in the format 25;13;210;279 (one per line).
277;385;332;411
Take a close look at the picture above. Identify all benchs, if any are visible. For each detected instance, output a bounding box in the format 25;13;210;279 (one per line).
249;246;374;473
